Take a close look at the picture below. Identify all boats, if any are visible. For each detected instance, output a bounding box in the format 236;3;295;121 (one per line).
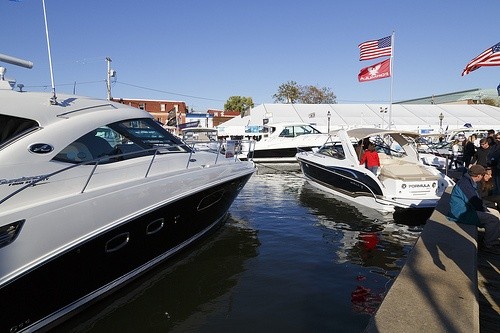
185;109;500;171
293;120;456;225
0;0;258;333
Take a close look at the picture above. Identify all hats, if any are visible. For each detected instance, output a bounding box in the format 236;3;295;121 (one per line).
468;165;488;177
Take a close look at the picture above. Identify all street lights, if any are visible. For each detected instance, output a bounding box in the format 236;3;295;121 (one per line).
326;111;332;134
439;112;445;129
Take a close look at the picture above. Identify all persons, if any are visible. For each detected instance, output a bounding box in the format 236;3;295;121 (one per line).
447;130;500;255
355;137;380;175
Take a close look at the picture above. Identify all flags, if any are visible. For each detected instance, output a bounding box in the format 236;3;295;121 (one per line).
462;41;500;76
358;58;390;82
358;36;391;61
